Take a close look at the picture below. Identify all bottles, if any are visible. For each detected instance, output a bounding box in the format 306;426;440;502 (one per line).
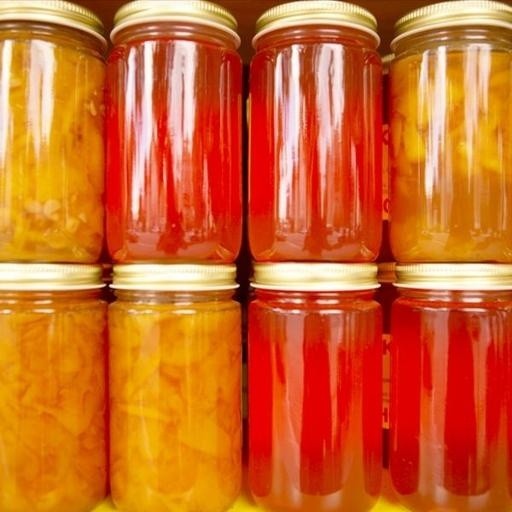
387;0;512;263
390;264;512;511
248;0;382;262
0;264;108;512
247;263;383;511
104;0;243;264
2;0;104;262
109;264;242;512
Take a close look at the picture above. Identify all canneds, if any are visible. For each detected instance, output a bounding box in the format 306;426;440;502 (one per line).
0;1;107;263
246;262;383;512
389;0;512;263
108;0;242;262
246;1;385;261
388;263;512;511
0;264;109;512
109;263;243;512
